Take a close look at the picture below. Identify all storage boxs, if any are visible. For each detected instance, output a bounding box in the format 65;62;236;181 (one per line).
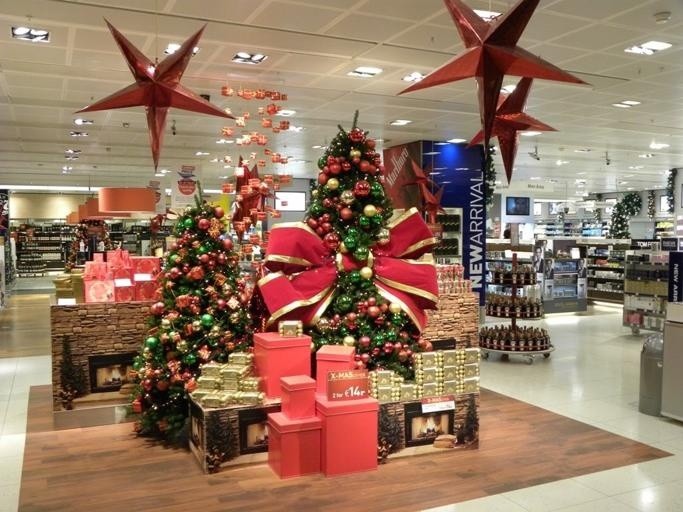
249;328;380;480
80;247;161;302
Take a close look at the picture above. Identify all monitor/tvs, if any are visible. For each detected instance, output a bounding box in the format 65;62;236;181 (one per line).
534;202;543;216
604;197;619;214
584;198;597;213
548;202;567;216
273;190;307;213
505;196;531;216
659;194;670;213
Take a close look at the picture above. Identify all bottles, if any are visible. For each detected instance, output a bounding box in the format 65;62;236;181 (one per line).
479;324;550;350
485;296;545;318
494;265;536;285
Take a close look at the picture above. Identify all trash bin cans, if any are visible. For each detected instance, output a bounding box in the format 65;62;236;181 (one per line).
638;334;664;416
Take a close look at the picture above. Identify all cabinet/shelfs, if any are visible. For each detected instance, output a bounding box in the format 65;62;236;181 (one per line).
479;213;674;363
14;219;175;277
